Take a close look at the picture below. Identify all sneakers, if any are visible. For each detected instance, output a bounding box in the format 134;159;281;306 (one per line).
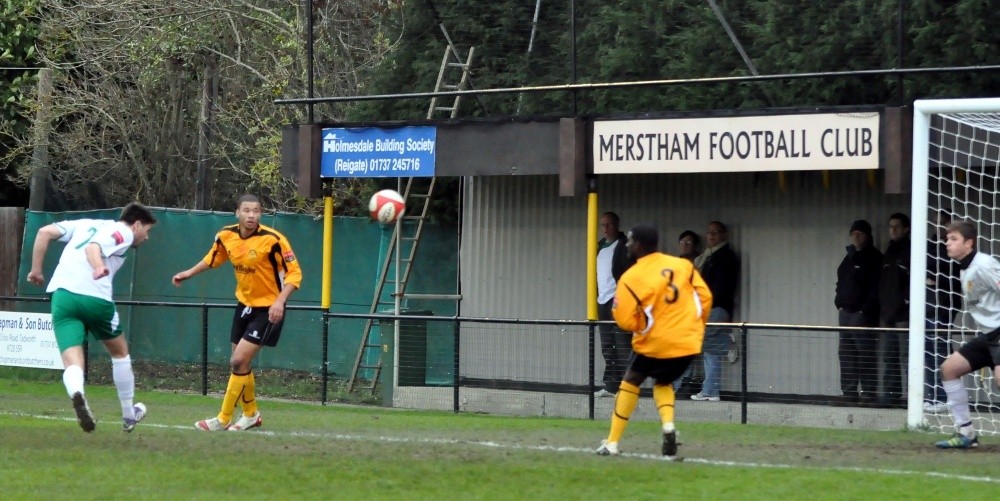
227;409;263;432
661;422;679;457
726;333;738;364
122;401;147;432
922;401;948;413
690;391;721;401
935;432;979;449
596;439;623;456
71;390;96;433
194;416;231;432
594;388;616;398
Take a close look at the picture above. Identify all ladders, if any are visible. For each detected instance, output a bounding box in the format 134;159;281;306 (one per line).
345;46;475;398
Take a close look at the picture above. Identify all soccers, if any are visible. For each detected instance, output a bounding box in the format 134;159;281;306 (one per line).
369;190;405;224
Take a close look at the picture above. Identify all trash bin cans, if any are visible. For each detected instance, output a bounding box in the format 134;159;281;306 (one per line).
376;309;431;409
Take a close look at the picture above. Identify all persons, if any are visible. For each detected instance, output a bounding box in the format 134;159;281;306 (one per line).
172;195;302;430
28;202;156;434
595;212;739;401
834;210;961;414
594;226;713;456
935;221;1000;448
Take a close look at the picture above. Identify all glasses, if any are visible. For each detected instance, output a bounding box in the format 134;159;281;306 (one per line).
680;239;694;246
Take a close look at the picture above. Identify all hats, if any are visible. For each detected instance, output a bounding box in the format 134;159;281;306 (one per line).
850;219;872;236
